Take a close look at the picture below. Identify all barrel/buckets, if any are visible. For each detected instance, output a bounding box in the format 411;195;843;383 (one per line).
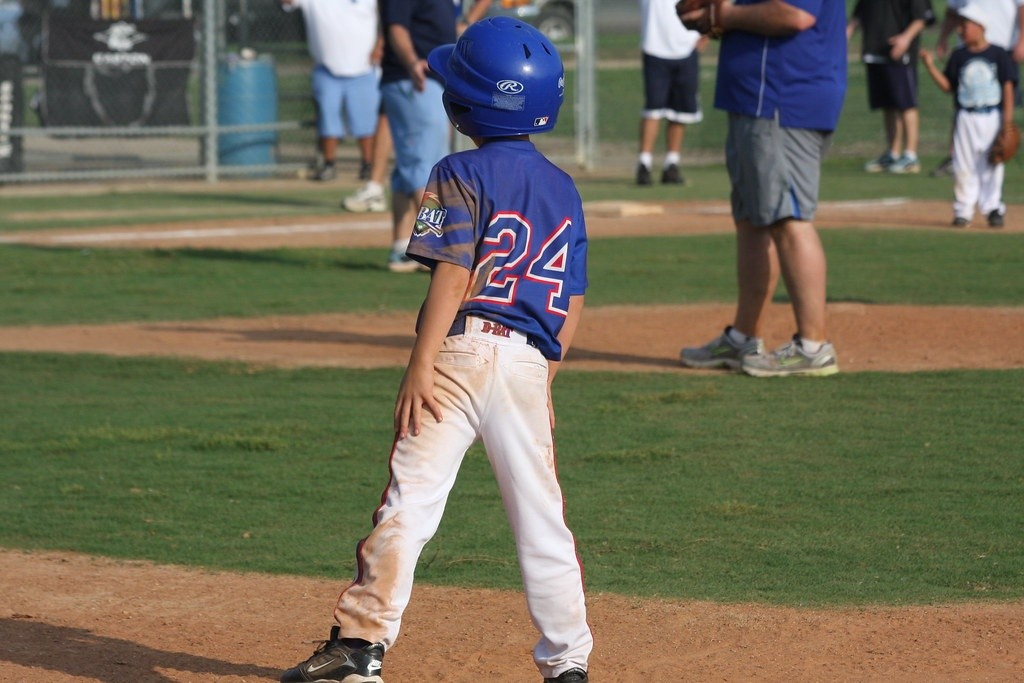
214;55;280;177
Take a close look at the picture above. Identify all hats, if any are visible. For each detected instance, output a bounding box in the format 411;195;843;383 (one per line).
958;4;988;27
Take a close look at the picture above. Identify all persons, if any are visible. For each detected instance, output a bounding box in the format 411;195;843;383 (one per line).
919;0;1024;228
634;0;711;185
845;0;936;174
675;0;847;376
279;16;593;683
282;1;518;272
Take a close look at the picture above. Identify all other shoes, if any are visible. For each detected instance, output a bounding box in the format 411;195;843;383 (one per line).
661;160;682;184
359;160;373;180
317;164;336;180
636;161;651;185
987;209;1004;227
953;217;970;227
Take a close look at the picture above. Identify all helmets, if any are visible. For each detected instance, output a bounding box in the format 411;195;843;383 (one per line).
428;16;565;136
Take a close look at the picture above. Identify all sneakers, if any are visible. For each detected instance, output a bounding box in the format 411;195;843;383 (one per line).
543;667;589;683
387;249;431;272
344;184;387;212
741;333;839;376
280;626;385;683
864;153;897;172
680;326;764;370
889;156;921;174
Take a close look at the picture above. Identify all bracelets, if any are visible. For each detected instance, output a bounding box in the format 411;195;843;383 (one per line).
463;18;470;27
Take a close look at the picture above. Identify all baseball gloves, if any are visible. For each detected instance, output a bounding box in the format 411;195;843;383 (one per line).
986;123;1019;168
674;0;726;40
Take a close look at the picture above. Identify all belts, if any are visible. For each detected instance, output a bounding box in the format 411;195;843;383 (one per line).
446;317;540;349
962;104;1000;114
